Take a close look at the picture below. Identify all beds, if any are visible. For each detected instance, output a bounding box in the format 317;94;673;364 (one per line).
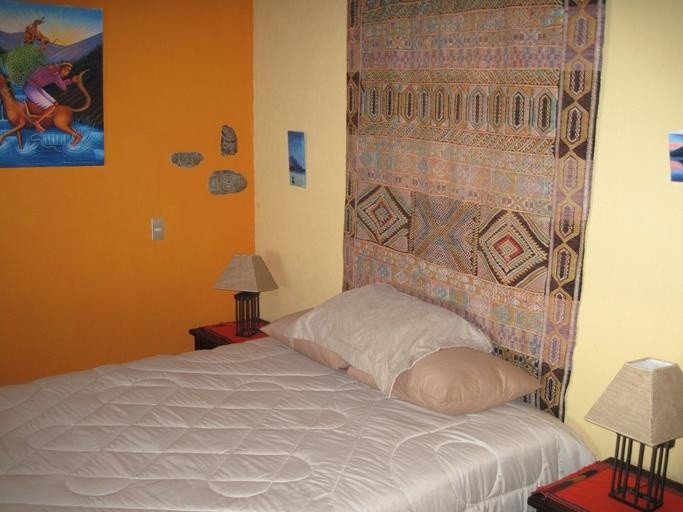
0;334;592;512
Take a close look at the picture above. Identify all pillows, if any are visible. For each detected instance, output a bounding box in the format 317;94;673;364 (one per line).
259;309;350;371
285;283;494;399
347;352;543;416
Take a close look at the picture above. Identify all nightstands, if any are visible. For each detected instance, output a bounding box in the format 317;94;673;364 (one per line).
527;456;683;512
189;318;270;350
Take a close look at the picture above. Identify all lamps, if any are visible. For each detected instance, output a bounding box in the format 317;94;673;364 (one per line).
583;357;683;512
212;254;278;338
